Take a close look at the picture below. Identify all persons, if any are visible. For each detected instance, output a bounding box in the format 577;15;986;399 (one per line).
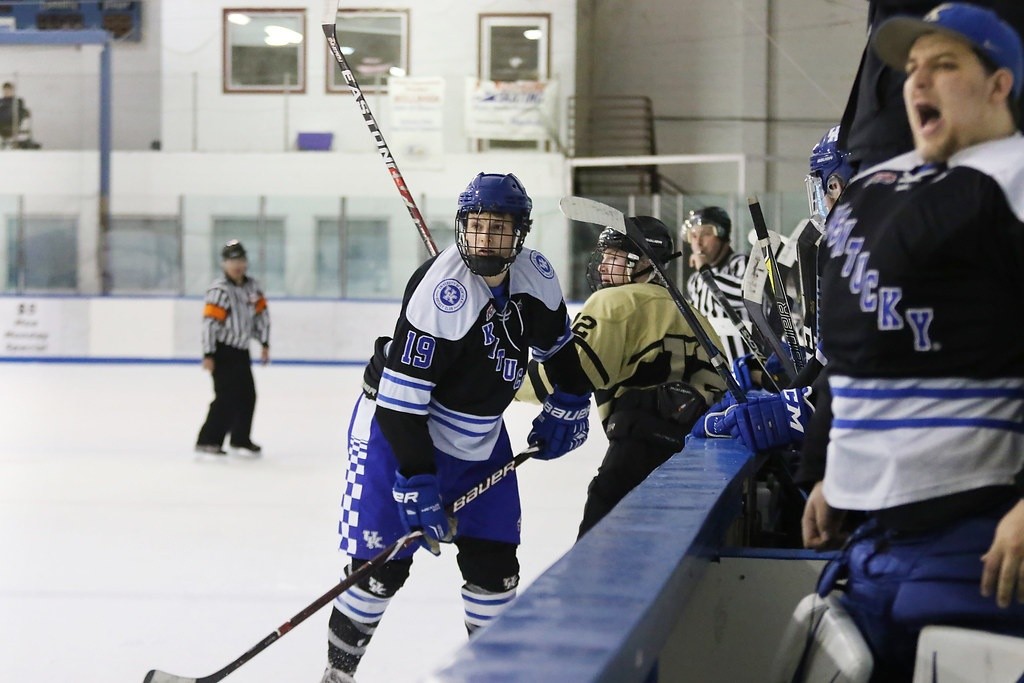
676;202;753;387
512;213;729;544
0;80;32;149
193;239;271;456
694;0;1024;683
316;167;592;683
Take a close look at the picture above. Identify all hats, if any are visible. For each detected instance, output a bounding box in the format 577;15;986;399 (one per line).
222;239;245;258
871;3;1024;103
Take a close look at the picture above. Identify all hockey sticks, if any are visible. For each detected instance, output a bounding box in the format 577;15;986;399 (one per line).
559;195;747;402
795;213;826;364
319;0;440;259
741;229;798;385
701;270;786;393
143;441;542;683
747;192;805;376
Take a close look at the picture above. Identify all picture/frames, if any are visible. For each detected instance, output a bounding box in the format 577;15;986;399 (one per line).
326;8;407;94
478;12;554;151
223;7;305;93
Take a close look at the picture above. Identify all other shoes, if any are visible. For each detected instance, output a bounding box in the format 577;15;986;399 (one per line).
194;443;227;455
322;666;358;683
230;435;260;453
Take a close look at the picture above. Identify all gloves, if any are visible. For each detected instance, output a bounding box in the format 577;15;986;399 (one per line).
390;467;459;556
733;353;763;390
714;391;813;453
526;387;591;461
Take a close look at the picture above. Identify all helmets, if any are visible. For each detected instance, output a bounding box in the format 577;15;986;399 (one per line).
455;172;532;277
685;207;733;242
585;216;674;294
804;124;855;223
